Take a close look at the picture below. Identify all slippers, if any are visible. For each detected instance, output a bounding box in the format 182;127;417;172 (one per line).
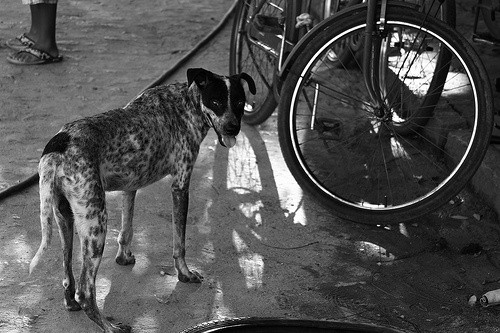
6;33;35;50
6;46;63;65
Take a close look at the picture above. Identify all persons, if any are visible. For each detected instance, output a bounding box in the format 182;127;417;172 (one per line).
0;0;64;67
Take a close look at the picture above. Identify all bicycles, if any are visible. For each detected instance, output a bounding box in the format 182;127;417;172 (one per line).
228;0;493;226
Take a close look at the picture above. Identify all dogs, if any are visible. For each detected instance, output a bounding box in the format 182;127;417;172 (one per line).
30;68;257;333
